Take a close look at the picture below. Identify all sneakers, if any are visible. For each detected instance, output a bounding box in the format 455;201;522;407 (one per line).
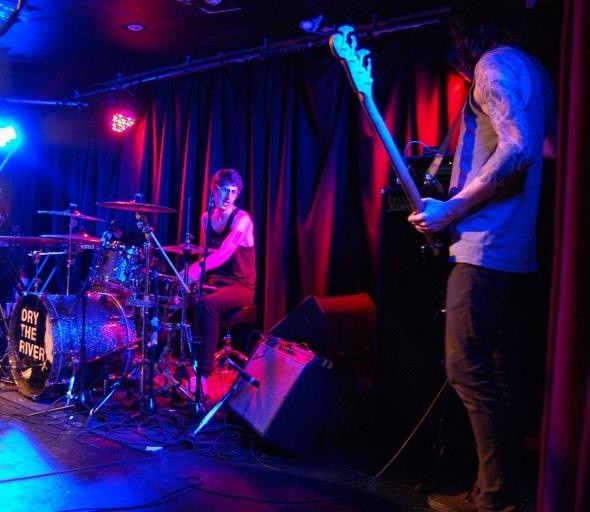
190;375;209;397
428;492;515;512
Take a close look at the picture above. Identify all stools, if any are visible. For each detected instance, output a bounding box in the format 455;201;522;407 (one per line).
214;303;258;361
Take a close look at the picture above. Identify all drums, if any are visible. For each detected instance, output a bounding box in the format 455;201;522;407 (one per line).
7;293;134;401
83;239;149;316
136;272;194;312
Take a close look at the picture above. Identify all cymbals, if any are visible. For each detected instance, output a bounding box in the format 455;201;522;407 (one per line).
40;232;102;244
95;199;179;214
0;235;67;244
37;211;106;222
154;243;218;255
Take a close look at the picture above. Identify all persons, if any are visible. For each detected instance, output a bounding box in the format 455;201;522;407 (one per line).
101;218;129;247
406;0;554;511
172;169;257;400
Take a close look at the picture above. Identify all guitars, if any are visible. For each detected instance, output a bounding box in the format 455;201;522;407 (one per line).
327;24;457;325
